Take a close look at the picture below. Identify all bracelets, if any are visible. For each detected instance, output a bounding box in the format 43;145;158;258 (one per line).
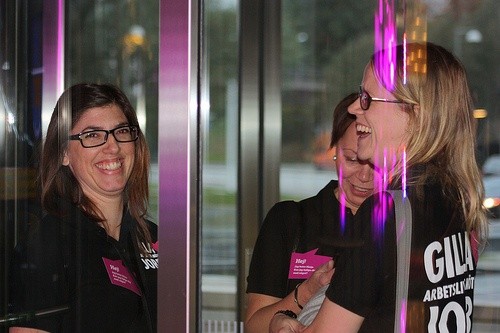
294;283;304;310
274;309;297;319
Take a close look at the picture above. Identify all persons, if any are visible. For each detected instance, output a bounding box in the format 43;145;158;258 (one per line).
268;42;489;333
244;92;382;333
9;83;159;333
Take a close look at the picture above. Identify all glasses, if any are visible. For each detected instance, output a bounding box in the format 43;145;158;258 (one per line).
68;125;139;148
359;85;410;110
